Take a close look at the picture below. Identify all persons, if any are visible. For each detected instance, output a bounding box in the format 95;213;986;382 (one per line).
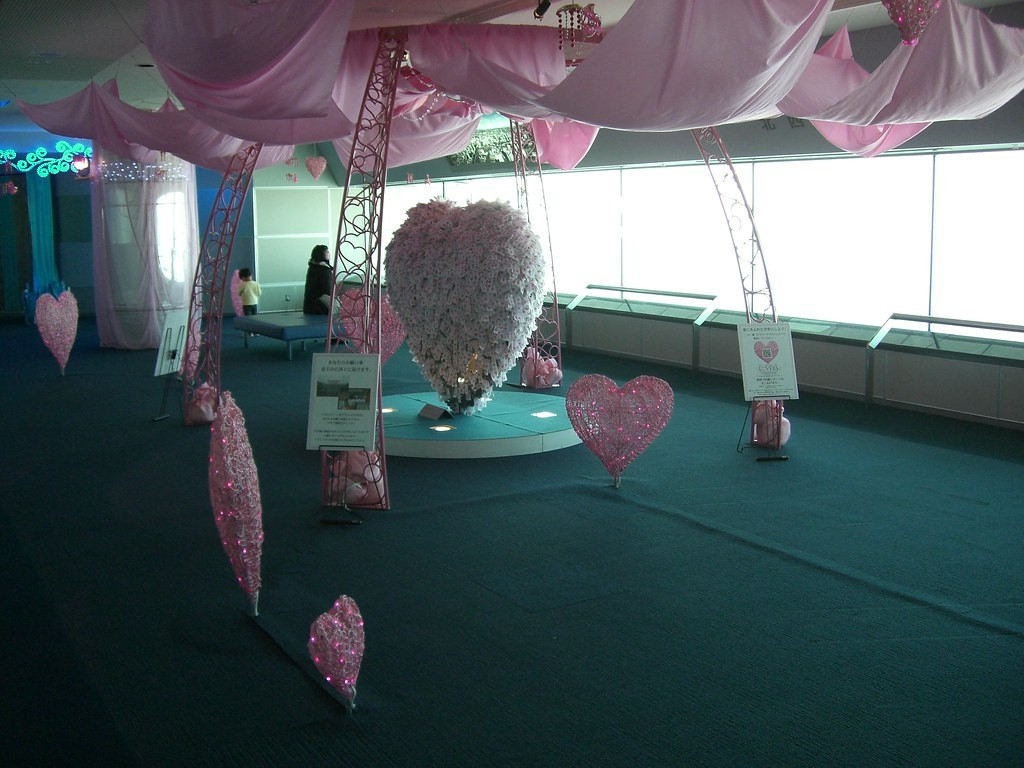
303;244;349;345
237;267;263;337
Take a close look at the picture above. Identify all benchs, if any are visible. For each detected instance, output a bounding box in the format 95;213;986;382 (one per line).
233;312;345;361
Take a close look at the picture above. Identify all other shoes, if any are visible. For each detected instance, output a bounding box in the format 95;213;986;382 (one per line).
255;334;260;336
249;333;254;337
325;338;349;345
